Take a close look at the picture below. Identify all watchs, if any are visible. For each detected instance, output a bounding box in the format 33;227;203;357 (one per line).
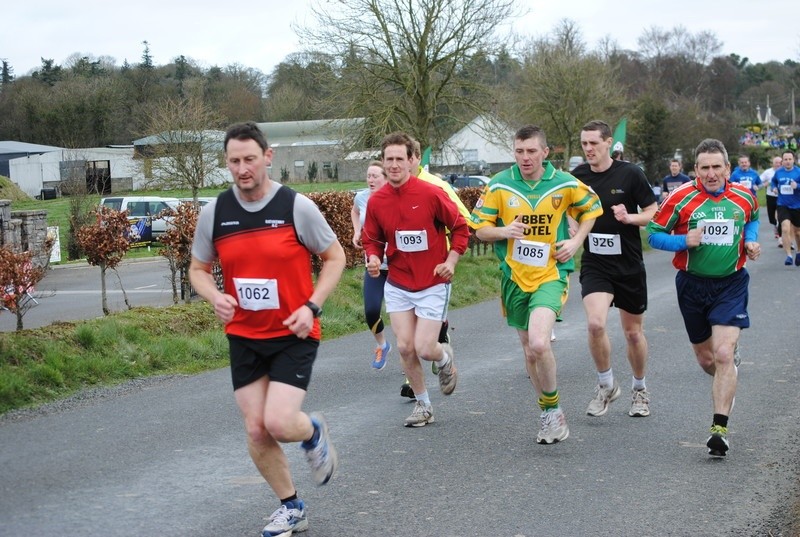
305;301;323;319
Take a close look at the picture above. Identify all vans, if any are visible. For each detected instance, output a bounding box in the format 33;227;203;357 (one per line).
99;196;184;238
177;197;214;211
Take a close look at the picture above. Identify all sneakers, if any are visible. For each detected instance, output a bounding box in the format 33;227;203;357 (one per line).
545;404;569;443
304;413;335;485
537;412;556;444
587;380;621;416
707;422;729;456
373;340;391;370
261;500;308;537
438;343;457;394
629;389;651;416
404;400;435;426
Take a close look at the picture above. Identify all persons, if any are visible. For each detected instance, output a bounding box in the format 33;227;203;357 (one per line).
469;125;603;445
350;129;471;429
611;150;800;265
569;120;659;417
188;121;347;537
647;139;762;457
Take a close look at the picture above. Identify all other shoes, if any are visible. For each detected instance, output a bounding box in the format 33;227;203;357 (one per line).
785;256;793;266
778;238;783;248
400;383;417;398
775;233;779;238
432;333;451;374
795;253;800;266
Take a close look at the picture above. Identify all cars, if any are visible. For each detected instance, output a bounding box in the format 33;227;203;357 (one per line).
443;175;492;188
